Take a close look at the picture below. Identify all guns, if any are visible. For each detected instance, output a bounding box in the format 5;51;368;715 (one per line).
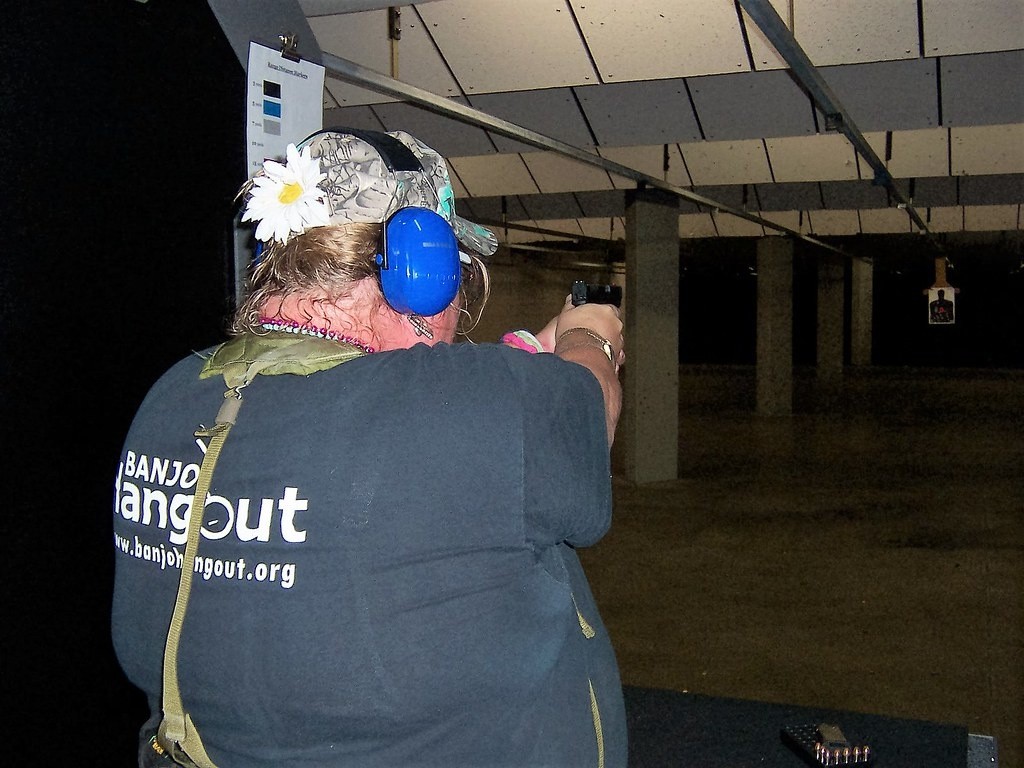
571;279;623;308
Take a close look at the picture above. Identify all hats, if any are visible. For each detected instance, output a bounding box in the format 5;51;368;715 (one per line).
242;127;497;256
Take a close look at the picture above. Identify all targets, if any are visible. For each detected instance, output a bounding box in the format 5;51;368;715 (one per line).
928;287;953;324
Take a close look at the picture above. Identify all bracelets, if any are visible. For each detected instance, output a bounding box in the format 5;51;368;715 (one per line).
498;330;544;354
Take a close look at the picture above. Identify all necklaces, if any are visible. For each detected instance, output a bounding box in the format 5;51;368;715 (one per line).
259;317;375;353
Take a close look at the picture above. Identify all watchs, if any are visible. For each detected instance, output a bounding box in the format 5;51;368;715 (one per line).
556;328;614;362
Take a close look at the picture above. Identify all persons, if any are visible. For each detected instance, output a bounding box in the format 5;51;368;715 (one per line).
111;126;629;768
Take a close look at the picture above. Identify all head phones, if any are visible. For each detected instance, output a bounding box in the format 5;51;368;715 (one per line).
254;126;461;318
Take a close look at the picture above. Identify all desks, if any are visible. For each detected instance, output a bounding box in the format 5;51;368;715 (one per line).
621;686;1000;768
